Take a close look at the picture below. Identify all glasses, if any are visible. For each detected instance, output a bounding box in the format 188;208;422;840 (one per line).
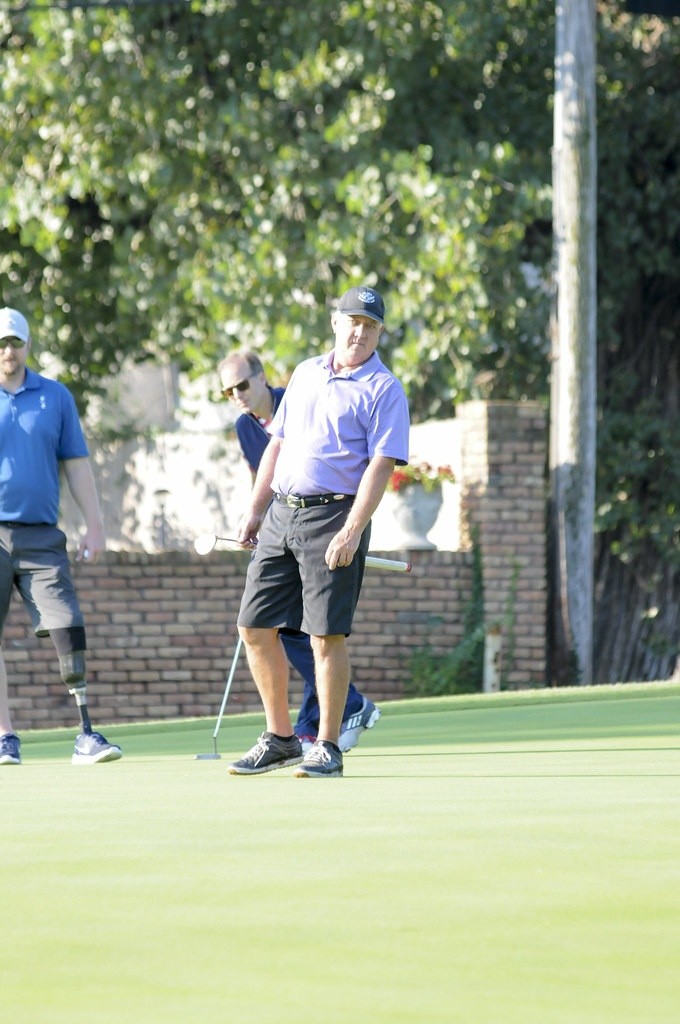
0;338;25;348
223;379;250;396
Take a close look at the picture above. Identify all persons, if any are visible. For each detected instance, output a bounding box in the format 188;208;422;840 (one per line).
220;354;382;755
226;286;408;777
0;305;123;765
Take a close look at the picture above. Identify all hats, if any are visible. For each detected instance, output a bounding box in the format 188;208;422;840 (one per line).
0;306;30;343
336;285;386;325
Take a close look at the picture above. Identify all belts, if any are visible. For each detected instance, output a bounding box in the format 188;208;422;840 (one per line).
274;492;349;508
0;520;55;530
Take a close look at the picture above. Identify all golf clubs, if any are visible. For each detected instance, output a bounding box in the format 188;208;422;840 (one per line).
194;530;412;574
196;637;242;760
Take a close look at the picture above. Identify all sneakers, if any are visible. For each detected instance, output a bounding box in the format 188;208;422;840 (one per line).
227;730;306;776
296;734;317;756
71;731;123;764
337;695;382;753
0;733;22;764
290;740;344;778
